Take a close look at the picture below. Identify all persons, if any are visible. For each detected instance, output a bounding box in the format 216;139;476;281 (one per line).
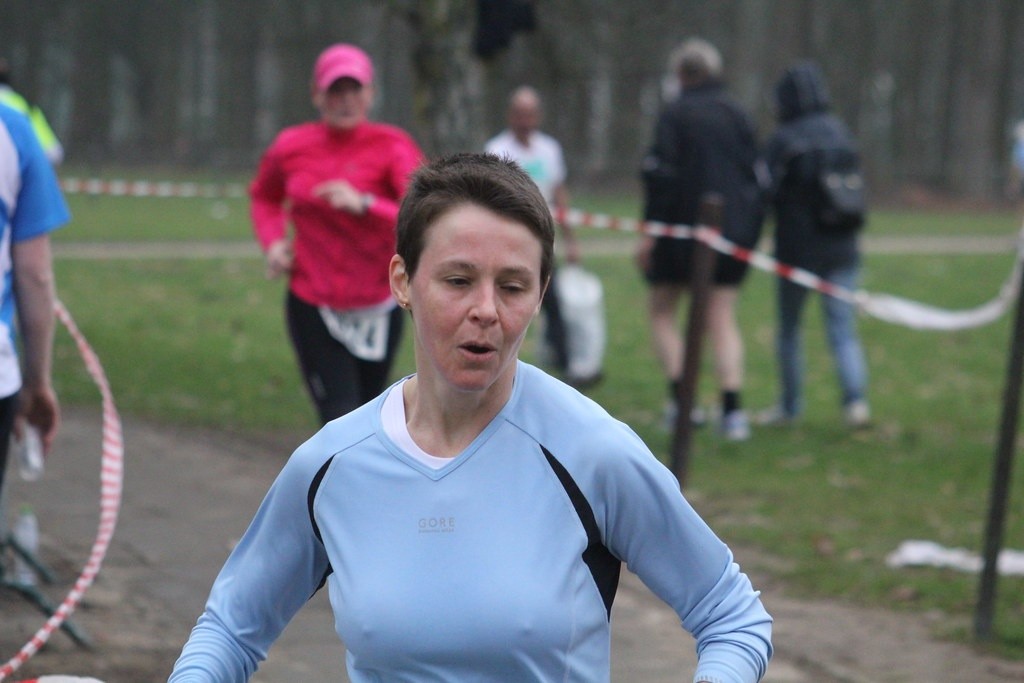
483;85;578;386
0;58;72;587
637;39;763;441
750;60;871;426
162;154;773;683
248;43;428;428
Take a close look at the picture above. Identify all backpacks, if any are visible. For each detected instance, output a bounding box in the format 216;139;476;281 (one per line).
779;122;871;236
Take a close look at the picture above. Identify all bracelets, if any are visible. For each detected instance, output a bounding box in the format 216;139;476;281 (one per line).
355;194;369;221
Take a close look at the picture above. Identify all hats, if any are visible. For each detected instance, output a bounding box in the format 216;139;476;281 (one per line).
313;43;372;91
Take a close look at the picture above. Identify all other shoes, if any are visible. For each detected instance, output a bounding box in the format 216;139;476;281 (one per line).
663;404;702;430
845;402;869;427
720;409;749;442
753;409;797;427
